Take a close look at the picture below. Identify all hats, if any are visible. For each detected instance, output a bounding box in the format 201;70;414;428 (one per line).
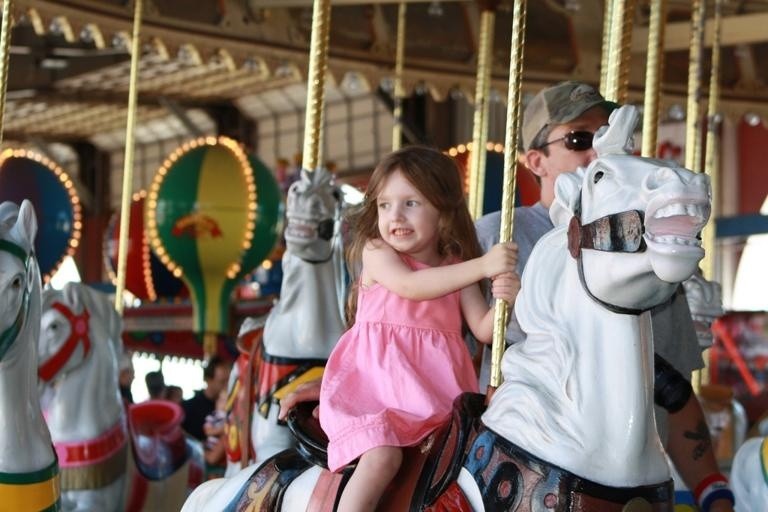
521;79;621;153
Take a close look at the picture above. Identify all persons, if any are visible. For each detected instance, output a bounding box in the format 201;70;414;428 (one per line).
178;355;233;468
652;353;734;512
317;143;521;512
145;367;166;408
166;385;183;402
119;367;135;404
278;76;739;512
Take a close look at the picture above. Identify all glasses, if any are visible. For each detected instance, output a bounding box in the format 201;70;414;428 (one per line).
536;130;594;152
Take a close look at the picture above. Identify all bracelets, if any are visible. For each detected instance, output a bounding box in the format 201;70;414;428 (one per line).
691;469;737;512
